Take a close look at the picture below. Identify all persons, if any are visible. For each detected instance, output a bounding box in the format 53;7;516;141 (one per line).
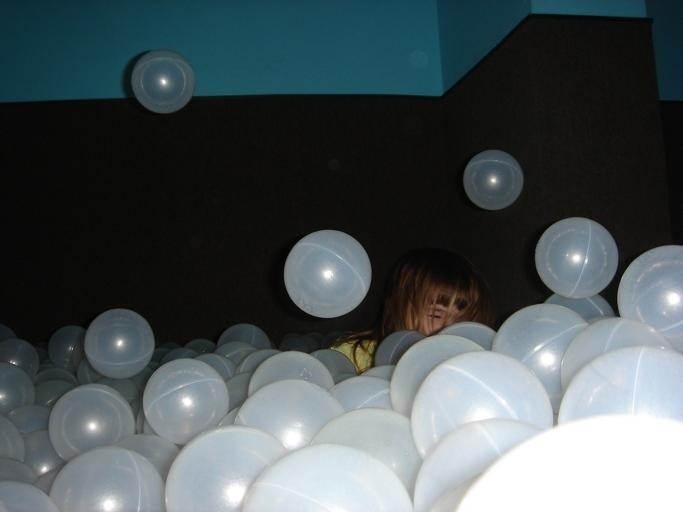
330;248;494;375
